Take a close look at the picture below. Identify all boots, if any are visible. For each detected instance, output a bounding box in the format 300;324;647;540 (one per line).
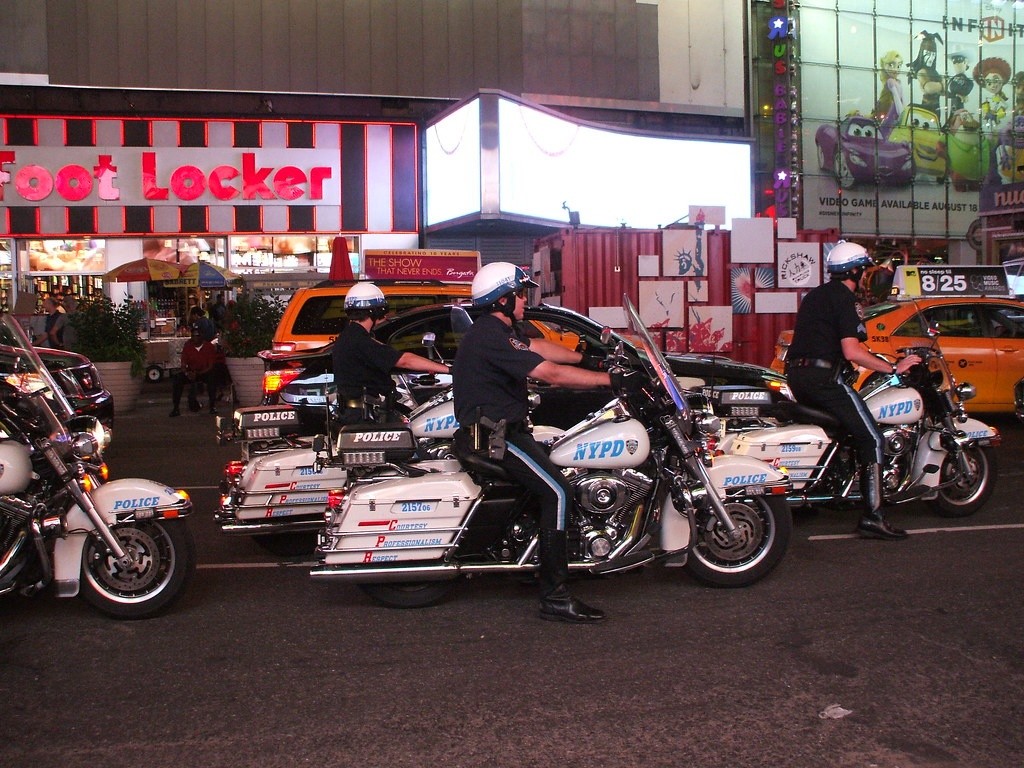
537;527;607;624
857;462;907;541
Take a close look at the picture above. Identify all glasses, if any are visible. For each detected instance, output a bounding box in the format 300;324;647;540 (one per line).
505;287;526;300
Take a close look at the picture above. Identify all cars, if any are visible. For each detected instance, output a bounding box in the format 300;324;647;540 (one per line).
769;261;1024;427
254;303;799;444
0;342;116;444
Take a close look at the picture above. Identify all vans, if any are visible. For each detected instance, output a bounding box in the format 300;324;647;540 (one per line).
272;248;647;366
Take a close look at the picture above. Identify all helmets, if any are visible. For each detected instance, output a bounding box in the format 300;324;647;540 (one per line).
827;239;877;272
344;283;386;313
471;262;540;307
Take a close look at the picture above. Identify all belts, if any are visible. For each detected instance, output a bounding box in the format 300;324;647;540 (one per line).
788;358;836;370
458;417;533;437
340;398;364;409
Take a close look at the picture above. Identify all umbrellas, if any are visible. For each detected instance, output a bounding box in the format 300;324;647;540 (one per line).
164;260;243;309
101;256;191;298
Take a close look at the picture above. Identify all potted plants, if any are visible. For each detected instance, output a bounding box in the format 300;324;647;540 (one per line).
67;285;148;412
217;293;286;406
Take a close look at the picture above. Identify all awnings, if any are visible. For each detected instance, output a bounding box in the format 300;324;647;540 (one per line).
242;273;330;288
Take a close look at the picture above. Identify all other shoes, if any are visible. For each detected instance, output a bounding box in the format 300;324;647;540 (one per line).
169;408;180;418
209;405;218;414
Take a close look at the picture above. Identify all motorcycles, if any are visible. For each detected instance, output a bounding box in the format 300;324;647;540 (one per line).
0;315;197;618
310;294;793;608
697;297;1004;518
209;307;568;559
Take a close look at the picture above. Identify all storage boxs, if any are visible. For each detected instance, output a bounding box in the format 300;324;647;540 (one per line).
704;384;773;420
235;404;302;430
341;421;420;464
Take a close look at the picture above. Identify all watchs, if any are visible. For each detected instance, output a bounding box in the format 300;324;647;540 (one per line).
888;363;897;375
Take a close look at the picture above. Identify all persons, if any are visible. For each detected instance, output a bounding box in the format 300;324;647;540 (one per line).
31;296;82;353
178;294;240;337
332;281;453;426
785;239;922;541
451;260;652;624
169;323;225;417
